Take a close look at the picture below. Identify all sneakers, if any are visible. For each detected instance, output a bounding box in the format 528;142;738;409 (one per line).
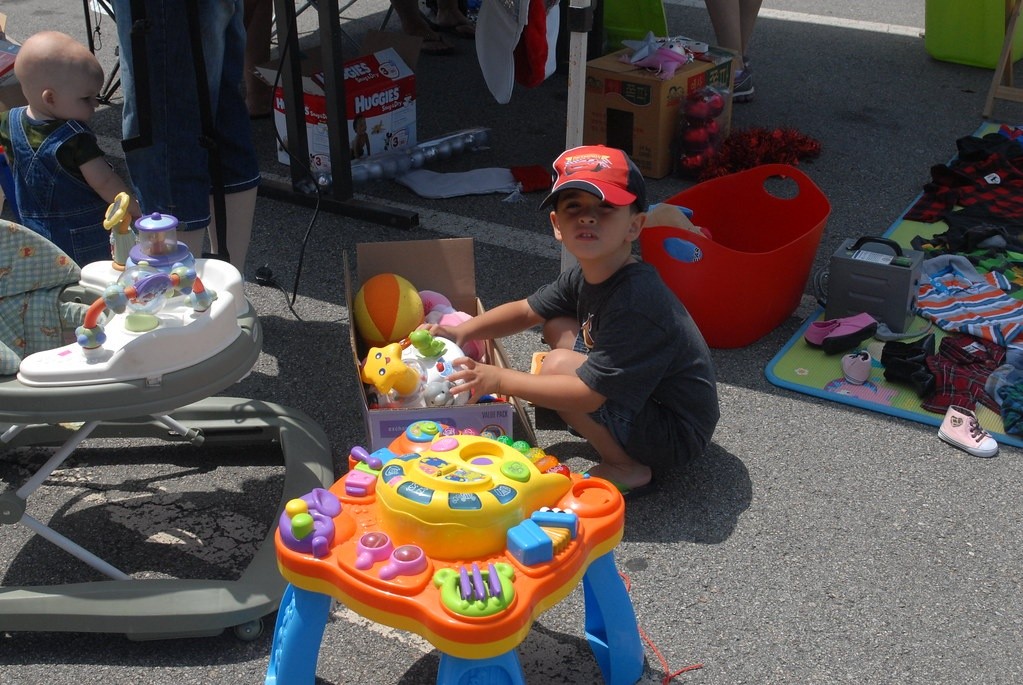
938;404;998;457
842;350;871;385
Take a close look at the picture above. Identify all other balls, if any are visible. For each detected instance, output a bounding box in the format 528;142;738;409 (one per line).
352;273;426;349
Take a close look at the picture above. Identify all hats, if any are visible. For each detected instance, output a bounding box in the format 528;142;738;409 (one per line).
538;144;646;211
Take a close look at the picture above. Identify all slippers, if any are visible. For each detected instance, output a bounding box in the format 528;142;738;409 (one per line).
420;32;455;55
433;18;475;38
577;467;653;500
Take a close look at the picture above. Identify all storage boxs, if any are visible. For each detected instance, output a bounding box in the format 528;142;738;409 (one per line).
341;238;540;452
582;46;737;179
250;27;426;171
640;164;830;348
925;0;1023;71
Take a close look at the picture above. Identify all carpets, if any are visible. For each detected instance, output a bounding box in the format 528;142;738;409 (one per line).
763;121;1023;447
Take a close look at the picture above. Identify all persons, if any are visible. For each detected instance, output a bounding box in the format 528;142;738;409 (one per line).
704;0;762;99
414;145;721;503
110;0;263;290
349;114;372;161
0;31;142;271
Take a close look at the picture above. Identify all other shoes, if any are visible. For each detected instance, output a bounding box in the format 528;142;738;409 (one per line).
804;318;837;347
823;312;878;355
732;68;754;101
